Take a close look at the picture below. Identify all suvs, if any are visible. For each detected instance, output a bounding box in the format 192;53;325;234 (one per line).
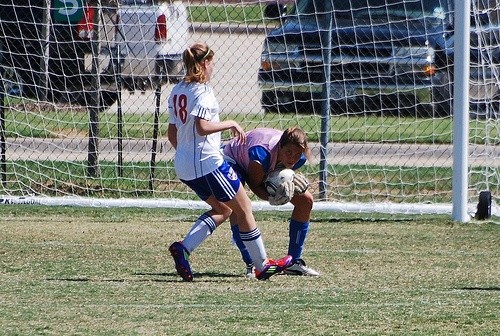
258;0;500;119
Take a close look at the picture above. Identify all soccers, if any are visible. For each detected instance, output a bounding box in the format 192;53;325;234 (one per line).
264;167;296;196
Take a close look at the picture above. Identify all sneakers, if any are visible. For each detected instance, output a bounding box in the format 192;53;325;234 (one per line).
246;264;256;277
169;243;193;281
284;258;320;276
254;255;292;280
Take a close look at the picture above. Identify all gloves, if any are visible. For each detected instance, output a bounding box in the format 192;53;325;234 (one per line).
292;172;308;195
268;180;294;206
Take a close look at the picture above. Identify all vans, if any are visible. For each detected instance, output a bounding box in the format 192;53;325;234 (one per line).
0;0;194;112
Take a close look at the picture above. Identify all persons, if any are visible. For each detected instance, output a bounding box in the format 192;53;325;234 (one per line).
221;126;319;279
168;44;293;281
